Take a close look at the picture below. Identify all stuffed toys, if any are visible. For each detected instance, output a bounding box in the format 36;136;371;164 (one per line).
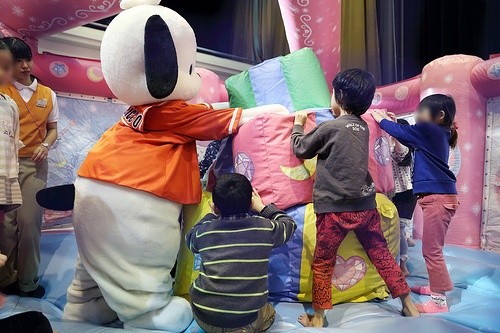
36;0;290;333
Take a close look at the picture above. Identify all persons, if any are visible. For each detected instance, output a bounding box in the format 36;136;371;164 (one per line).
369;94;461;313
0;37;60;299
290;68;421;327
389;119;417;277
184;173;297;333
0;40;26;309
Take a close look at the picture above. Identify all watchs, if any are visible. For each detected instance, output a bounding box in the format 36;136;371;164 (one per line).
39;143;50;150
